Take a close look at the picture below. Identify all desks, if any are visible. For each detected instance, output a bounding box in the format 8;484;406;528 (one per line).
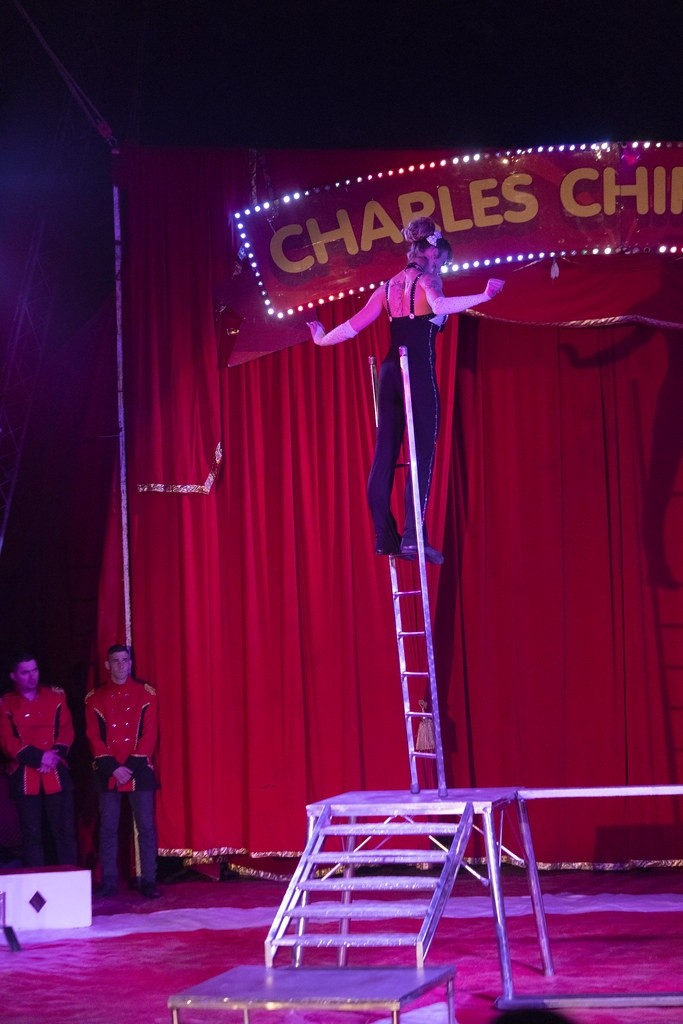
168;964;454;1024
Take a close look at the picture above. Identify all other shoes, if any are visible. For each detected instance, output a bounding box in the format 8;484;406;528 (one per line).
399;538;444;565
139;883;160;899
375;536;403;556
98;887;117;897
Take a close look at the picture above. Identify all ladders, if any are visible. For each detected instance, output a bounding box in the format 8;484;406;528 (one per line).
367;343;450;800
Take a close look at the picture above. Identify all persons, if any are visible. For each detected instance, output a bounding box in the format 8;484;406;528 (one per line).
0;654;78;868
86;643;162;898
306;217;505;565
